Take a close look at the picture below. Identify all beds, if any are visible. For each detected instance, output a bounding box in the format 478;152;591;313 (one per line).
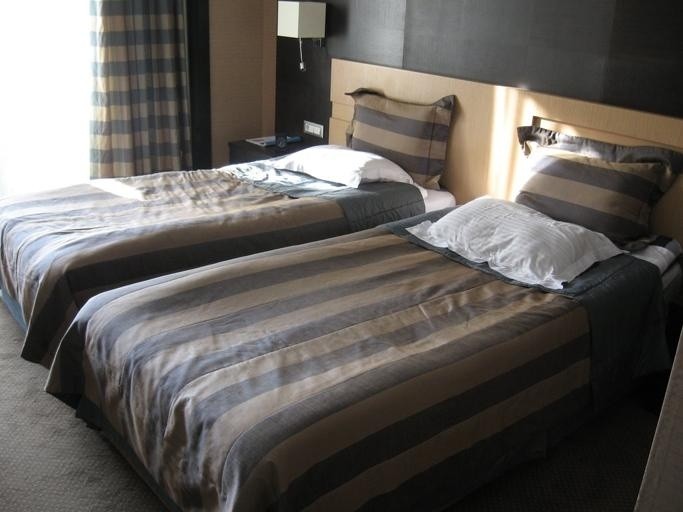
0;54;525;372
44;115;681;509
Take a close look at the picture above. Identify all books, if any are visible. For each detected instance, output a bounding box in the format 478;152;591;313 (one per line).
245;134;302;147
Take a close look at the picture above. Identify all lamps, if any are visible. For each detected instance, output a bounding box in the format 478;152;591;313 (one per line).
276;1;327;73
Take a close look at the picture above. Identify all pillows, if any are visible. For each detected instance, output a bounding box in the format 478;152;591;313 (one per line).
340;87;455;189
514;126;683;239
272;144;417;189
406;195;629;291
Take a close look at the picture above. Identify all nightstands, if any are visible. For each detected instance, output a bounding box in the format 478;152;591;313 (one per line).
226;136;313;162
